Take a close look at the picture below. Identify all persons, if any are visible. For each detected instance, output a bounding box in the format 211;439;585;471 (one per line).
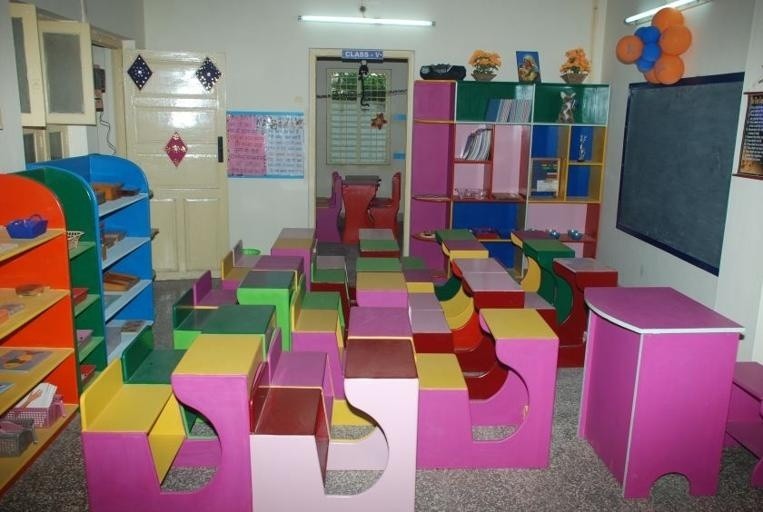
518;55;539;83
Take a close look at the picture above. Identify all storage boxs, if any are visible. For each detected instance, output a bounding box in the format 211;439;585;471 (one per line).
0;418;34;457
4;394;64;428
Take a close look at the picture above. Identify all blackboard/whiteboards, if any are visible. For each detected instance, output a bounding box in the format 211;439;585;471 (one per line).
616;72;746;277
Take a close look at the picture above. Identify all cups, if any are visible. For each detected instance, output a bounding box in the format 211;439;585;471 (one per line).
424;230;432;235
568;229;578;238
572;232;582;240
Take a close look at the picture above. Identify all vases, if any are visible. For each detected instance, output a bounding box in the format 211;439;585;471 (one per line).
561;74;590;83
472;73;497;81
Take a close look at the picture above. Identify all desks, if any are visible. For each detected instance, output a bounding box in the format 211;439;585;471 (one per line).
342;175;381;244
577;285;746;498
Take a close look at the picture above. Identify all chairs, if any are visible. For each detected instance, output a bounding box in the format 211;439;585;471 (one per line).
723;362;763;489
368;171;401;236
316;171;342;244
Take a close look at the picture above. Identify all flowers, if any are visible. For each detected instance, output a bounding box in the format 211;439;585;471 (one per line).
560;49;592;73
469;50;501;73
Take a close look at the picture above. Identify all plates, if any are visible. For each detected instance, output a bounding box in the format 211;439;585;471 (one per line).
413;231;437;241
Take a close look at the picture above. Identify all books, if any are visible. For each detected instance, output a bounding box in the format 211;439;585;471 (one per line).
0;381;14;395
461;128;493;161
120;319;146;335
0;348;54;375
485;98;533;123
6;382;58;425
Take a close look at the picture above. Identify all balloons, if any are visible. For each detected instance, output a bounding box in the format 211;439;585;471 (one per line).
616;7;693;85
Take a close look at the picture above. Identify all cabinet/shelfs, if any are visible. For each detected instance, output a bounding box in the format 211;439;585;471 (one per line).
409;79;612;280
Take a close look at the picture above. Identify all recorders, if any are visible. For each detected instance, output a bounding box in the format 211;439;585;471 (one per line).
420;64;466;80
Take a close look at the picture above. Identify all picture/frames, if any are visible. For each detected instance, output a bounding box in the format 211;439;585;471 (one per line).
530;157;561;199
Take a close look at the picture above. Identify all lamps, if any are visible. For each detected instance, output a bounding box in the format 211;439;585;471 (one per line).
623;0;710;26
298;15;436;27
